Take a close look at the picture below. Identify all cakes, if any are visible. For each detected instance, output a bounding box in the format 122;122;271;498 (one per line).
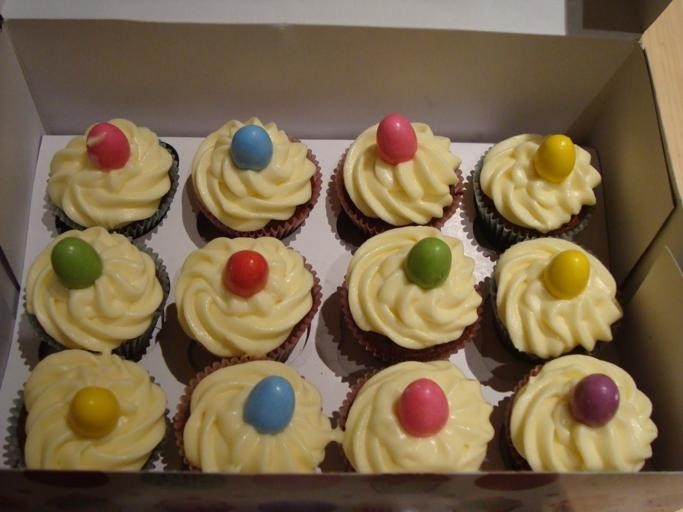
480;132;603;235
190;115;316;232
342;225;482;349
175;237;315;356
22;349;168;472
343;112;462;227
24;226;164;354
343;359;495;474
182;360;331;472
46;117;173;229
493;236;624;360
501;354;658;474
